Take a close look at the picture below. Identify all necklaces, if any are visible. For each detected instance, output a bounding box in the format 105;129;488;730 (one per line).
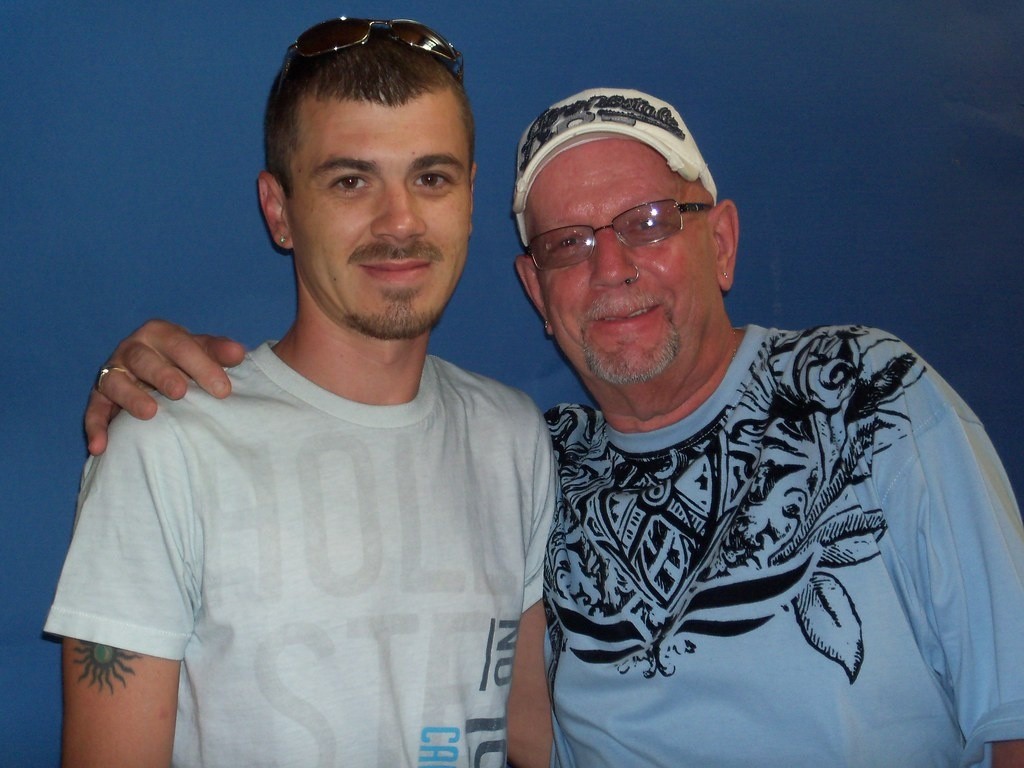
731;326;739;361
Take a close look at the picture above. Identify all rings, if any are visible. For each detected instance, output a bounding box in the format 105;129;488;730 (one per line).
98;366;128;392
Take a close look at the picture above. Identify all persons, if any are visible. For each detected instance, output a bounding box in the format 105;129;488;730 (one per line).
44;17;558;768
83;87;1024;768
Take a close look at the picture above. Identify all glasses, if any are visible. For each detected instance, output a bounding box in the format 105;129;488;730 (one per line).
524;199;713;271
275;17;464;105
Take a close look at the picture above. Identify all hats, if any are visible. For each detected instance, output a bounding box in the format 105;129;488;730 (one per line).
513;87;717;245
